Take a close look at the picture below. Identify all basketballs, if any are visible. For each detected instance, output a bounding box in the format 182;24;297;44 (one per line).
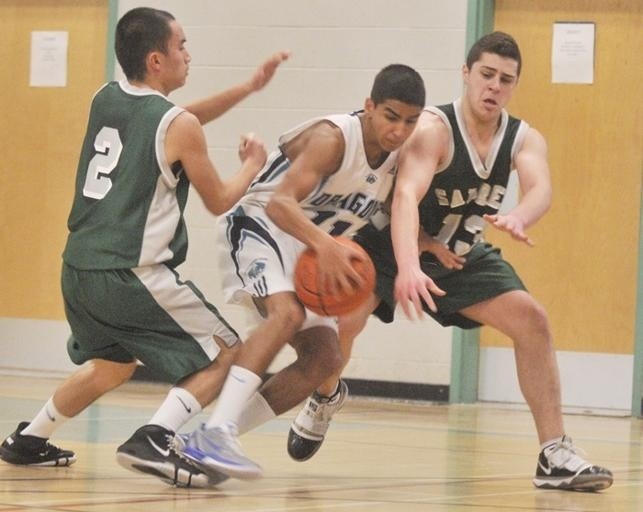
293;237;376;318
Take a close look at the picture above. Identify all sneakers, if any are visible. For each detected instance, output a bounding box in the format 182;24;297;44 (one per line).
287;379;349;463
0;422;77;469
115;424;265;489
533;435;613;491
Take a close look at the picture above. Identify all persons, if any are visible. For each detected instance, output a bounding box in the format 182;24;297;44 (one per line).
0;5;296;493
168;60;471;483
282;27;618;495
